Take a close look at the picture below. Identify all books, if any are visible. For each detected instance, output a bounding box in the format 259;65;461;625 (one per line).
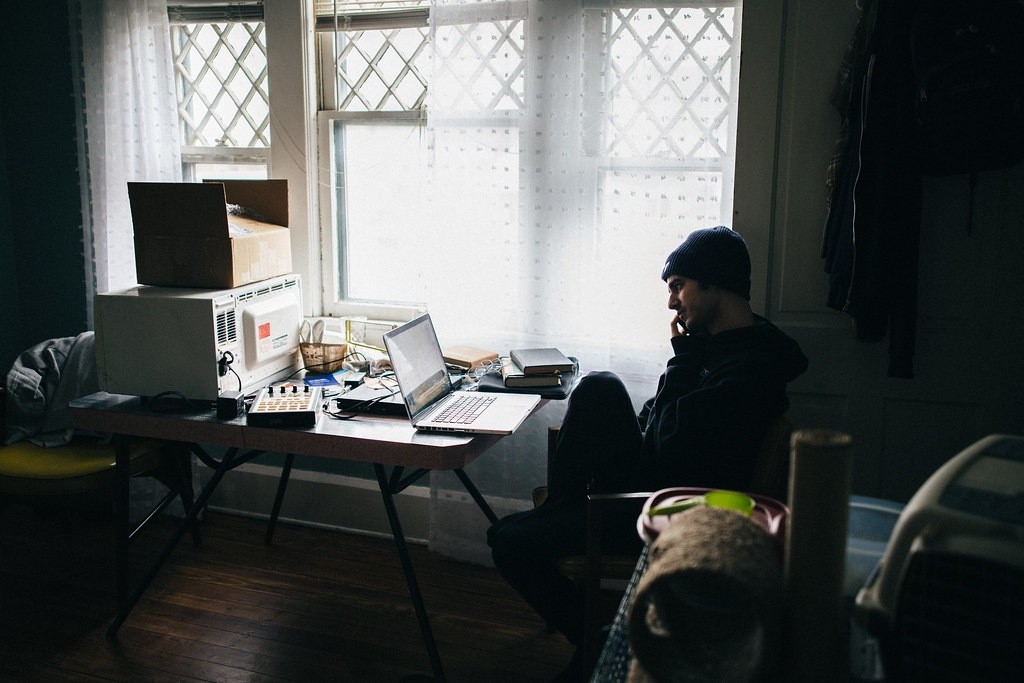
441;346;498;372
500;347;574;388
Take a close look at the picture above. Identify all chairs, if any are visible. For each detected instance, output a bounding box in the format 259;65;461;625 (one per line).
0;331;199;608
562;395;793;682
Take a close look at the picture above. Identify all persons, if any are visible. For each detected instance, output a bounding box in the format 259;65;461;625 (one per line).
486;225;808;683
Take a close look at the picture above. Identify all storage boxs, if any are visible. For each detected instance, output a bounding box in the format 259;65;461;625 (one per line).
126;180;291;288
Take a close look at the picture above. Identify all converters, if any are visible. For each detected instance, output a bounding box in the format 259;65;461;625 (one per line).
344;372;367;390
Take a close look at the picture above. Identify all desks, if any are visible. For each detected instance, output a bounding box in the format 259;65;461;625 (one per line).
70;390;551;683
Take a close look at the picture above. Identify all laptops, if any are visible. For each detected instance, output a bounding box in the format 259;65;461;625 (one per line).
382;313;539;434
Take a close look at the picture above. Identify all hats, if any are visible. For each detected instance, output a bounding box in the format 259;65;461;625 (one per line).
661;226;751;300
